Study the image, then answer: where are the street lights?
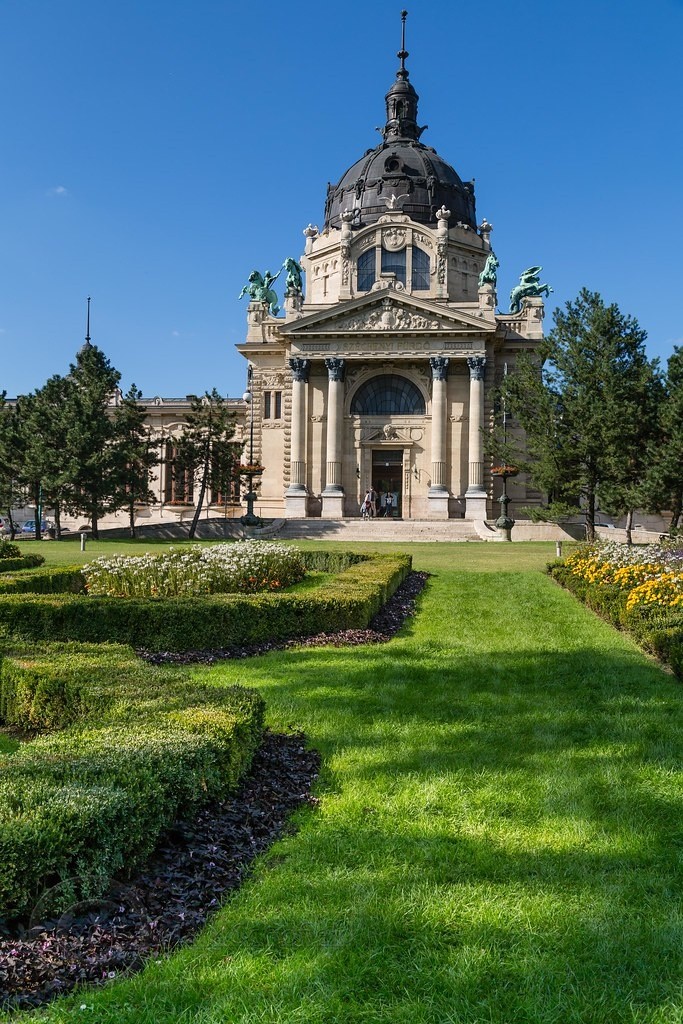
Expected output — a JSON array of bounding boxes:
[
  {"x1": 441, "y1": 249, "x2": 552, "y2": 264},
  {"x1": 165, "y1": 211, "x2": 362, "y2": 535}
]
[{"x1": 242, "y1": 364, "x2": 260, "y2": 526}]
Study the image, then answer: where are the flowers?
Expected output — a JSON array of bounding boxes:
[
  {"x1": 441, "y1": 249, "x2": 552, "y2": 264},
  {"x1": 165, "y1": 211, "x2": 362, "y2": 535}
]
[
  {"x1": 490, "y1": 466, "x2": 517, "y2": 476},
  {"x1": 210, "y1": 500, "x2": 242, "y2": 506},
  {"x1": 240, "y1": 464, "x2": 266, "y2": 471},
  {"x1": 162, "y1": 500, "x2": 195, "y2": 506}
]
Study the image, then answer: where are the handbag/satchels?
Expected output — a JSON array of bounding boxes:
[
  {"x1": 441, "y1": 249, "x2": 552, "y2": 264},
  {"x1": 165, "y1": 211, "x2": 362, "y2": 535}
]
[{"x1": 366, "y1": 501, "x2": 371, "y2": 508}]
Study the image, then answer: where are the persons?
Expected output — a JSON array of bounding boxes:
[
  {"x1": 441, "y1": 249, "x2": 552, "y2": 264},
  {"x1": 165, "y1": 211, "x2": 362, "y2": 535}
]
[
  {"x1": 361, "y1": 490, "x2": 370, "y2": 517},
  {"x1": 384, "y1": 491, "x2": 394, "y2": 517},
  {"x1": 264, "y1": 270, "x2": 281, "y2": 288},
  {"x1": 369, "y1": 486, "x2": 378, "y2": 516}
]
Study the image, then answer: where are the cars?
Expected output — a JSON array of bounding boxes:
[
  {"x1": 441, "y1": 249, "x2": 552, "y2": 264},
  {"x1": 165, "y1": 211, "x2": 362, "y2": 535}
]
[
  {"x1": 0, "y1": 517, "x2": 23, "y2": 535},
  {"x1": 22, "y1": 520, "x2": 55, "y2": 532}
]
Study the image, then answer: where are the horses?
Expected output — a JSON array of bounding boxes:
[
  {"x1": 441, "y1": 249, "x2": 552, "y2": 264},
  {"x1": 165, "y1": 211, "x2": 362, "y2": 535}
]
[
  {"x1": 239, "y1": 271, "x2": 278, "y2": 315},
  {"x1": 509, "y1": 266, "x2": 550, "y2": 312},
  {"x1": 282, "y1": 258, "x2": 302, "y2": 297},
  {"x1": 478, "y1": 250, "x2": 499, "y2": 289}
]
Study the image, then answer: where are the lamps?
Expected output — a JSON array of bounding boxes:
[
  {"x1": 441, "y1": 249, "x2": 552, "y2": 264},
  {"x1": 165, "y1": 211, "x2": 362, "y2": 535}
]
[
  {"x1": 355, "y1": 468, "x2": 360, "y2": 478},
  {"x1": 414, "y1": 469, "x2": 418, "y2": 479}
]
